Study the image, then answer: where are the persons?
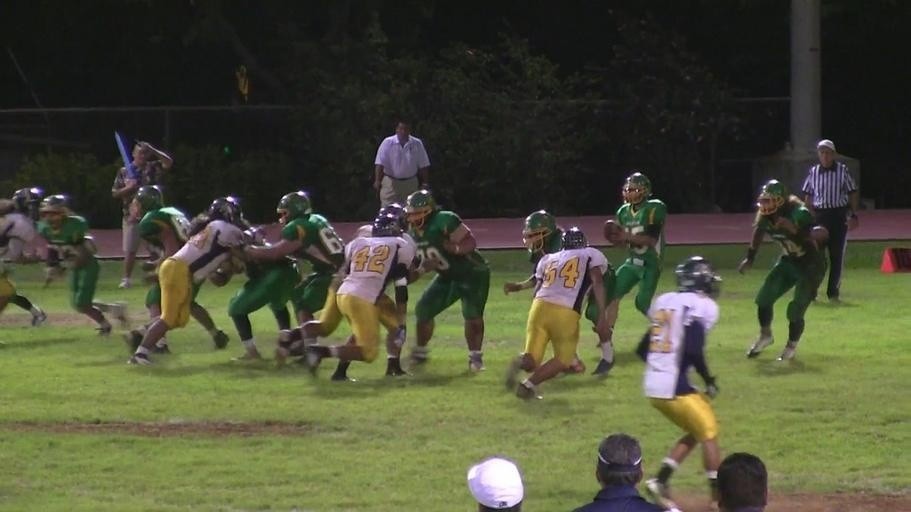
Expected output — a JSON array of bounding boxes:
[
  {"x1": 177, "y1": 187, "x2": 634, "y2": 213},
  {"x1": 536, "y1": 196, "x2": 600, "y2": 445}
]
[
  {"x1": 584, "y1": 171, "x2": 668, "y2": 356},
  {"x1": 375, "y1": 118, "x2": 433, "y2": 212},
  {"x1": 1, "y1": 186, "x2": 128, "y2": 335},
  {"x1": 573, "y1": 432, "x2": 668, "y2": 511},
  {"x1": 737, "y1": 178, "x2": 830, "y2": 362},
  {"x1": 636, "y1": 253, "x2": 722, "y2": 511},
  {"x1": 111, "y1": 140, "x2": 175, "y2": 289},
  {"x1": 504, "y1": 209, "x2": 612, "y2": 402},
  {"x1": 805, "y1": 139, "x2": 859, "y2": 304},
  {"x1": 675, "y1": 502, "x2": 678, "y2": 503},
  {"x1": 122, "y1": 185, "x2": 490, "y2": 382},
  {"x1": 715, "y1": 452, "x2": 769, "y2": 511}
]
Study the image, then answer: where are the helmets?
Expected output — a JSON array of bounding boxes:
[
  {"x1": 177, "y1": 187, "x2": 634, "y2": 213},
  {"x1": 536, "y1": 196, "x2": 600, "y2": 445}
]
[
  {"x1": 406, "y1": 188, "x2": 436, "y2": 216},
  {"x1": 206, "y1": 195, "x2": 244, "y2": 229},
  {"x1": 625, "y1": 170, "x2": 654, "y2": 200},
  {"x1": 133, "y1": 184, "x2": 165, "y2": 212},
  {"x1": 674, "y1": 254, "x2": 717, "y2": 295},
  {"x1": 370, "y1": 202, "x2": 410, "y2": 238},
  {"x1": 762, "y1": 177, "x2": 790, "y2": 207},
  {"x1": 276, "y1": 190, "x2": 311, "y2": 229},
  {"x1": 523, "y1": 208, "x2": 557, "y2": 243},
  {"x1": 563, "y1": 225, "x2": 588, "y2": 250},
  {"x1": 11, "y1": 186, "x2": 71, "y2": 222}
]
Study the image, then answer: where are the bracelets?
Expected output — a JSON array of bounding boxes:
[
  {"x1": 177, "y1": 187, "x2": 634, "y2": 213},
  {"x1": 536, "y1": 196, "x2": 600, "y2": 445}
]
[{"x1": 850, "y1": 212, "x2": 858, "y2": 220}]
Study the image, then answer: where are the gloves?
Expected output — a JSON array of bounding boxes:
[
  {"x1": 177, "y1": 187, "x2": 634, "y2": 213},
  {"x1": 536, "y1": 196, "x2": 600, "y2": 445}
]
[
  {"x1": 737, "y1": 246, "x2": 758, "y2": 275},
  {"x1": 702, "y1": 374, "x2": 721, "y2": 401},
  {"x1": 502, "y1": 280, "x2": 526, "y2": 297},
  {"x1": 408, "y1": 239, "x2": 465, "y2": 275}
]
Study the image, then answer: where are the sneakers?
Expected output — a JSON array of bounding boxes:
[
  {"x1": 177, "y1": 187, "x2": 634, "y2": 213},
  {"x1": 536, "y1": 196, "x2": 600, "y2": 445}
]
[
  {"x1": 502, "y1": 338, "x2": 617, "y2": 401},
  {"x1": 776, "y1": 341, "x2": 798, "y2": 361},
  {"x1": 114, "y1": 299, "x2": 131, "y2": 331},
  {"x1": 233, "y1": 349, "x2": 259, "y2": 360},
  {"x1": 382, "y1": 368, "x2": 413, "y2": 378},
  {"x1": 94, "y1": 323, "x2": 112, "y2": 338},
  {"x1": 468, "y1": 353, "x2": 481, "y2": 373},
  {"x1": 708, "y1": 499, "x2": 723, "y2": 512},
  {"x1": 118, "y1": 276, "x2": 132, "y2": 290},
  {"x1": 409, "y1": 347, "x2": 427, "y2": 364},
  {"x1": 808, "y1": 296, "x2": 845, "y2": 307},
  {"x1": 273, "y1": 329, "x2": 356, "y2": 382},
  {"x1": 126, "y1": 329, "x2": 143, "y2": 348},
  {"x1": 746, "y1": 330, "x2": 774, "y2": 359},
  {"x1": 212, "y1": 331, "x2": 229, "y2": 349},
  {"x1": 154, "y1": 339, "x2": 168, "y2": 352},
  {"x1": 126, "y1": 354, "x2": 155, "y2": 367},
  {"x1": 26, "y1": 307, "x2": 48, "y2": 331},
  {"x1": 642, "y1": 476, "x2": 680, "y2": 512}
]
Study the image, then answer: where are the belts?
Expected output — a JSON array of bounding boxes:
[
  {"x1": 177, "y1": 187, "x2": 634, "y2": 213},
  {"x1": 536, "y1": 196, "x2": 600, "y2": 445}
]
[{"x1": 383, "y1": 174, "x2": 418, "y2": 181}]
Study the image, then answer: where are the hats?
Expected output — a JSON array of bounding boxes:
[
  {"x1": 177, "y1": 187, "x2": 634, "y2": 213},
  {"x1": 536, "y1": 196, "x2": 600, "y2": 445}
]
[{"x1": 816, "y1": 138, "x2": 837, "y2": 155}]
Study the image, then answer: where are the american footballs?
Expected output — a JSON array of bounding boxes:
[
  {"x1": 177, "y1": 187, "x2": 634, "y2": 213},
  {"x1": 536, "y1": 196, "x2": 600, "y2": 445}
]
[{"x1": 602, "y1": 220, "x2": 621, "y2": 244}]
